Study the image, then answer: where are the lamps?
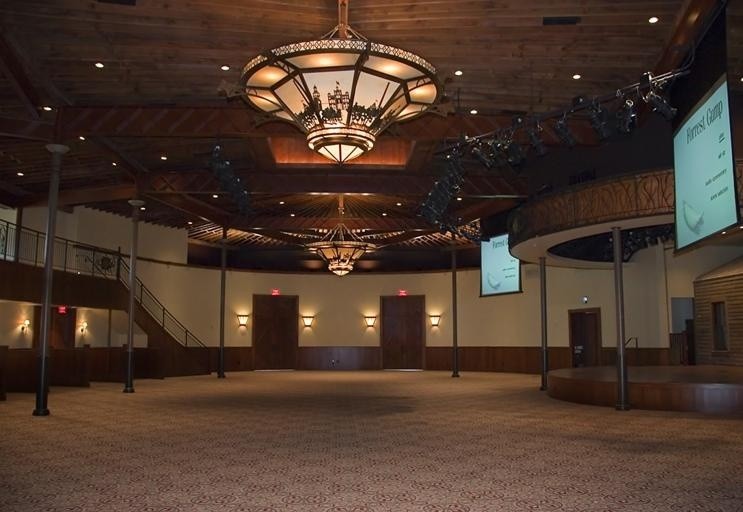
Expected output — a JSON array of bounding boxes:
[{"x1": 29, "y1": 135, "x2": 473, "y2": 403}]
[
  {"x1": 429, "y1": 314, "x2": 440, "y2": 327},
  {"x1": 581, "y1": 296, "x2": 589, "y2": 305},
  {"x1": 303, "y1": 192, "x2": 377, "y2": 277},
  {"x1": 237, "y1": 314, "x2": 249, "y2": 327},
  {"x1": 365, "y1": 317, "x2": 376, "y2": 327},
  {"x1": 19, "y1": 320, "x2": 30, "y2": 335},
  {"x1": 238, "y1": 12, "x2": 446, "y2": 168},
  {"x1": 302, "y1": 316, "x2": 315, "y2": 327},
  {"x1": 80, "y1": 321, "x2": 88, "y2": 333}
]
